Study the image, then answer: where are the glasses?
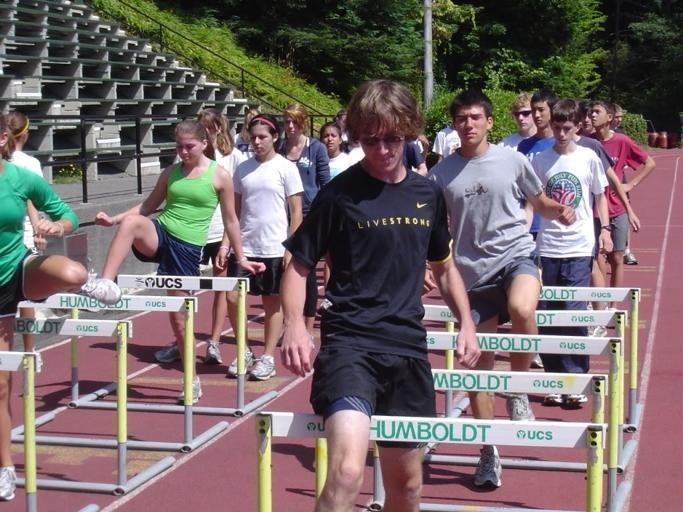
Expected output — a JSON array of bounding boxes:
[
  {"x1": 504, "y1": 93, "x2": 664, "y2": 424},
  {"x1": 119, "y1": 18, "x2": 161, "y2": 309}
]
[
  {"x1": 361, "y1": 133, "x2": 403, "y2": 148},
  {"x1": 514, "y1": 111, "x2": 532, "y2": 116}
]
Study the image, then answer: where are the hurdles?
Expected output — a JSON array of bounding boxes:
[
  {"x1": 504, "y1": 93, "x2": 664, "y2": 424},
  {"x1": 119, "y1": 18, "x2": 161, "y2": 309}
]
[
  {"x1": 10, "y1": 316, "x2": 178, "y2": 495},
  {"x1": 368, "y1": 330, "x2": 635, "y2": 512},
  {"x1": 537, "y1": 284, "x2": 642, "y2": 435},
  {"x1": 1, "y1": 350, "x2": 101, "y2": 512},
  {"x1": 420, "y1": 310, "x2": 638, "y2": 474},
  {"x1": 310, "y1": 365, "x2": 610, "y2": 511},
  {"x1": 69, "y1": 273, "x2": 277, "y2": 419},
  {"x1": 10, "y1": 293, "x2": 231, "y2": 455},
  {"x1": 422, "y1": 301, "x2": 473, "y2": 419},
  {"x1": 255, "y1": 409, "x2": 606, "y2": 512}
]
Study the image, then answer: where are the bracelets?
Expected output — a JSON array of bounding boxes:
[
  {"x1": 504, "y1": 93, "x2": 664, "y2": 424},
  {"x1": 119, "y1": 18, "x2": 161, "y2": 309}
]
[{"x1": 55, "y1": 222, "x2": 67, "y2": 241}]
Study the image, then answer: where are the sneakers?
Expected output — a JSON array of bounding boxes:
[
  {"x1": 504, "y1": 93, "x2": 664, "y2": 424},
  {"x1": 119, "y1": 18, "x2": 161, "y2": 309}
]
[
  {"x1": 0, "y1": 465, "x2": 18, "y2": 501},
  {"x1": 319, "y1": 297, "x2": 333, "y2": 311},
  {"x1": 278, "y1": 332, "x2": 315, "y2": 350},
  {"x1": 623, "y1": 251, "x2": 637, "y2": 265},
  {"x1": 505, "y1": 393, "x2": 534, "y2": 423},
  {"x1": 71, "y1": 271, "x2": 121, "y2": 304},
  {"x1": 586, "y1": 326, "x2": 607, "y2": 338},
  {"x1": 205, "y1": 338, "x2": 223, "y2": 363},
  {"x1": 155, "y1": 342, "x2": 183, "y2": 363},
  {"x1": 543, "y1": 390, "x2": 587, "y2": 406},
  {"x1": 531, "y1": 354, "x2": 545, "y2": 368},
  {"x1": 228, "y1": 347, "x2": 276, "y2": 381},
  {"x1": 474, "y1": 442, "x2": 502, "y2": 488},
  {"x1": 178, "y1": 375, "x2": 203, "y2": 404}
]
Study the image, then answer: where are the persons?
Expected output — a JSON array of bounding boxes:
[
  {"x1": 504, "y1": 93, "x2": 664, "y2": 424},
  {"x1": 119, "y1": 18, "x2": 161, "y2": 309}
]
[
  {"x1": 402, "y1": 88, "x2": 657, "y2": 491},
  {"x1": 279, "y1": 78, "x2": 481, "y2": 512},
  {"x1": 96, "y1": 105, "x2": 365, "y2": 404},
  {"x1": 4, "y1": 112, "x2": 47, "y2": 259},
  {"x1": 0, "y1": 122, "x2": 121, "y2": 504}
]
